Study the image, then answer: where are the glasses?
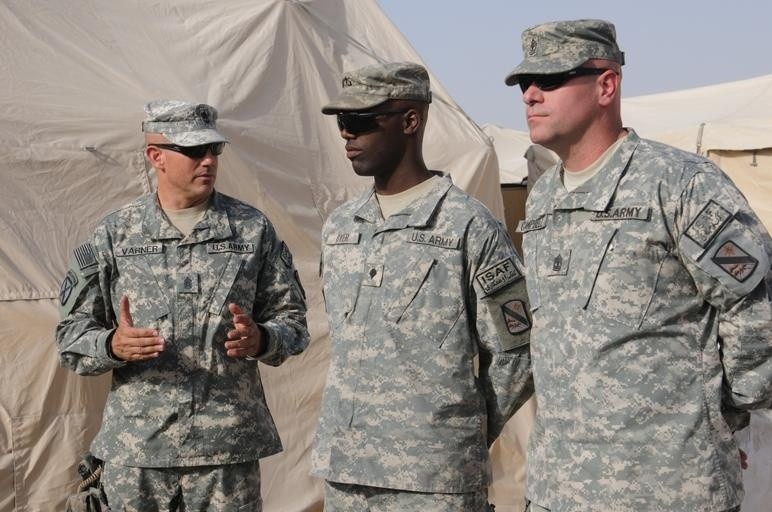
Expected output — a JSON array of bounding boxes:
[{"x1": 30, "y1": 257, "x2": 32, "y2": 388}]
[
  {"x1": 520, "y1": 67, "x2": 620, "y2": 95},
  {"x1": 337, "y1": 109, "x2": 425, "y2": 134},
  {"x1": 148, "y1": 143, "x2": 225, "y2": 158}
]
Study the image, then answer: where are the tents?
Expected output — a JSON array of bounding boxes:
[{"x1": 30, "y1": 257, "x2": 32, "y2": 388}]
[
  {"x1": 478, "y1": 121, "x2": 541, "y2": 264},
  {"x1": 0, "y1": 0, "x2": 533, "y2": 512},
  {"x1": 617, "y1": 73, "x2": 772, "y2": 242}
]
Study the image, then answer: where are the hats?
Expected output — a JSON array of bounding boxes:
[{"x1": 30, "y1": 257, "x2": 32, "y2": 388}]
[
  {"x1": 321, "y1": 61, "x2": 433, "y2": 115},
  {"x1": 141, "y1": 98, "x2": 231, "y2": 147},
  {"x1": 504, "y1": 19, "x2": 626, "y2": 87}
]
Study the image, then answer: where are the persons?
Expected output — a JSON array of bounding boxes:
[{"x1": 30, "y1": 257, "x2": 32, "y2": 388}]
[
  {"x1": 50, "y1": 96, "x2": 313, "y2": 512},
  {"x1": 303, "y1": 58, "x2": 535, "y2": 512},
  {"x1": 506, "y1": 15, "x2": 771, "y2": 512}
]
